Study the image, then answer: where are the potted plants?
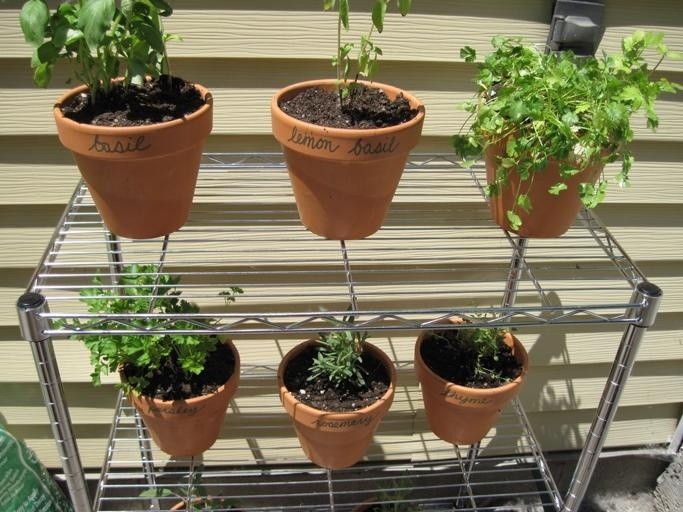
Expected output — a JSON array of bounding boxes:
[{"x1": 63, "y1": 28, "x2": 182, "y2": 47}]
[
  {"x1": 445, "y1": 27, "x2": 682, "y2": 243},
  {"x1": 348, "y1": 471, "x2": 421, "y2": 512},
  {"x1": 275, "y1": 300, "x2": 397, "y2": 468},
  {"x1": 19, "y1": 0, "x2": 215, "y2": 240},
  {"x1": 52, "y1": 263, "x2": 241, "y2": 457},
  {"x1": 412, "y1": 300, "x2": 530, "y2": 444},
  {"x1": 268, "y1": 1, "x2": 427, "y2": 241},
  {"x1": 137, "y1": 467, "x2": 242, "y2": 510}
]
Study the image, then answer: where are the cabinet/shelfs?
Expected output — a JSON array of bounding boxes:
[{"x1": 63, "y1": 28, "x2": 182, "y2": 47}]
[{"x1": 13, "y1": 152, "x2": 664, "y2": 510}]
